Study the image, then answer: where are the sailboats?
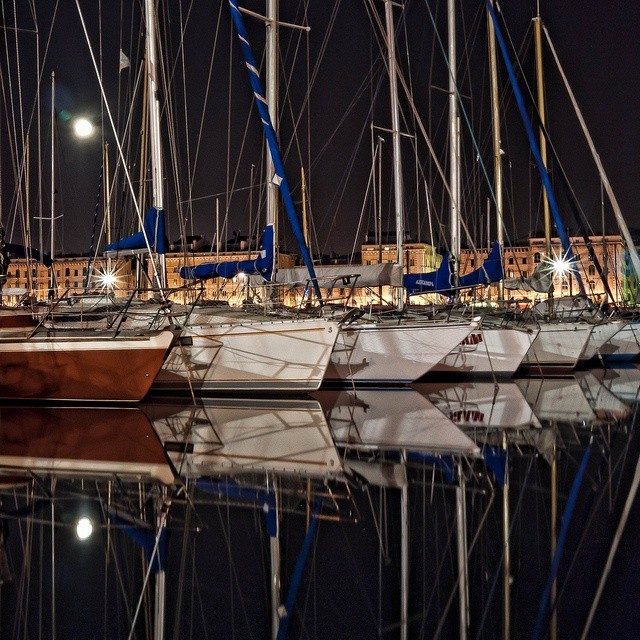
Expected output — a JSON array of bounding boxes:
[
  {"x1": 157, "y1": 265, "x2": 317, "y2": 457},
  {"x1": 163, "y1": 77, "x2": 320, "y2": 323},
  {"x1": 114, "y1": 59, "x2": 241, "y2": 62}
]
[
  {"x1": 1, "y1": 0, "x2": 640, "y2": 408},
  {"x1": 0, "y1": 362, "x2": 640, "y2": 638},
  {"x1": 2, "y1": 367, "x2": 638, "y2": 639}
]
